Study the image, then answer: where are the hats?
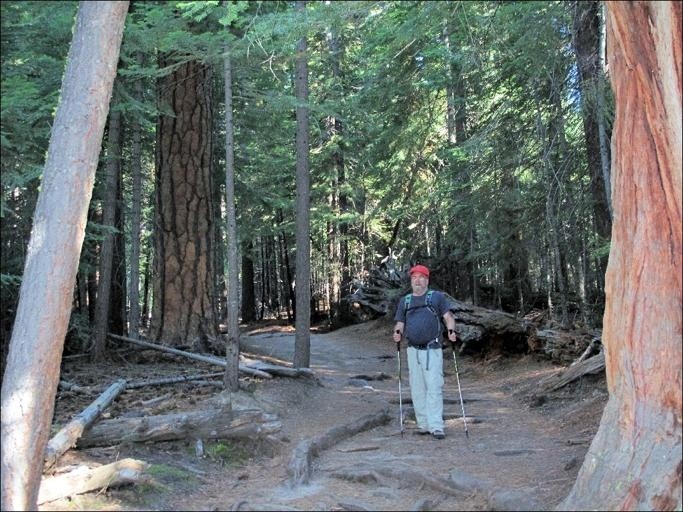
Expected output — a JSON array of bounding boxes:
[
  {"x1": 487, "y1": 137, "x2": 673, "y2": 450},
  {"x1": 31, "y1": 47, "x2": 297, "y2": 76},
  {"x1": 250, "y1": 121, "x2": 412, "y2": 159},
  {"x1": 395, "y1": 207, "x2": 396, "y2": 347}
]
[{"x1": 410, "y1": 265, "x2": 429, "y2": 276}]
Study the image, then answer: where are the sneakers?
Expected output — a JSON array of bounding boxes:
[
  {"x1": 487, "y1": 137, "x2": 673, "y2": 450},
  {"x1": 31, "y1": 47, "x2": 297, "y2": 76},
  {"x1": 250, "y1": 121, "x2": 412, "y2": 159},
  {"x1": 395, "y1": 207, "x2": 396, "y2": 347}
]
[
  {"x1": 433, "y1": 430, "x2": 445, "y2": 439},
  {"x1": 413, "y1": 427, "x2": 430, "y2": 435}
]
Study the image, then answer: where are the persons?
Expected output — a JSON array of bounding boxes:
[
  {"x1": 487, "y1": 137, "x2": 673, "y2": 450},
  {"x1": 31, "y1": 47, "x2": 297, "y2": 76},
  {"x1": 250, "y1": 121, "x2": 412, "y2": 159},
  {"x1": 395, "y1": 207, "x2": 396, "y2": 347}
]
[{"x1": 392, "y1": 265, "x2": 457, "y2": 440}]
